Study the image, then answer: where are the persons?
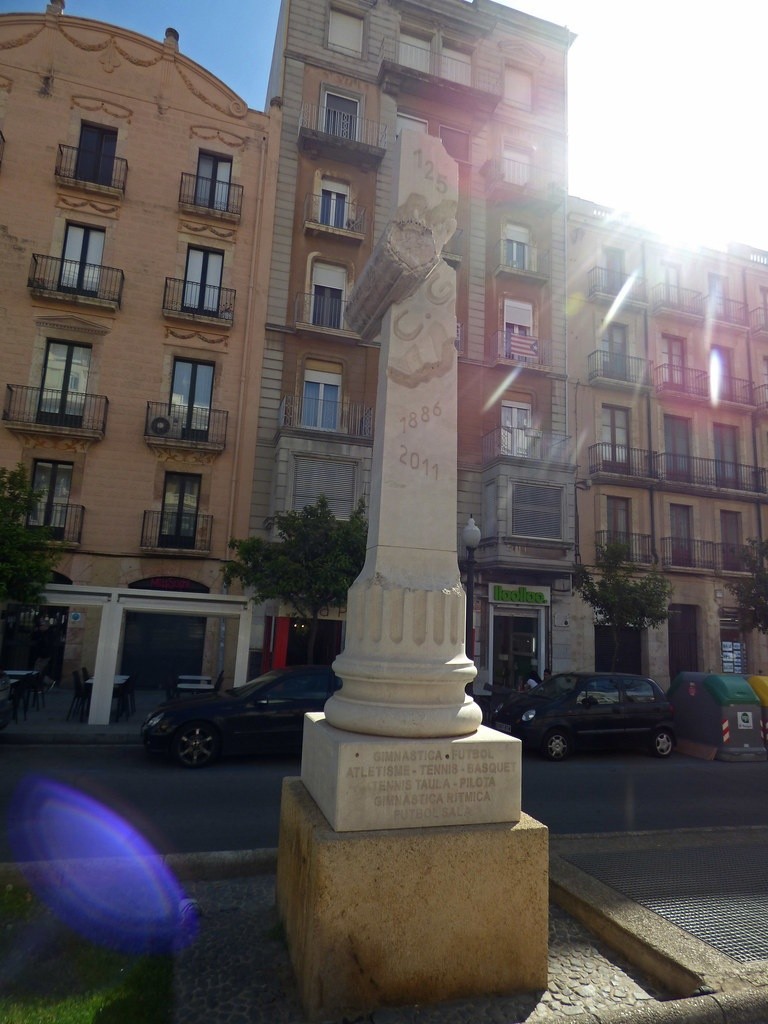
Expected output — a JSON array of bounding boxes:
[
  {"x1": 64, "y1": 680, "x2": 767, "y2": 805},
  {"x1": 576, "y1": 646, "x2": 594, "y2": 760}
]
[{"x1": 525, "y1": 671, "x2": 542, "y2": 692}]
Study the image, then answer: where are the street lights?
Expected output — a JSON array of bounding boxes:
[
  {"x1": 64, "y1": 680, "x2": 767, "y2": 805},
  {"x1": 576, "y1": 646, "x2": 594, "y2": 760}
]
[{"x1": 462, "y1": 513, "x2": 480, "y2": 698}]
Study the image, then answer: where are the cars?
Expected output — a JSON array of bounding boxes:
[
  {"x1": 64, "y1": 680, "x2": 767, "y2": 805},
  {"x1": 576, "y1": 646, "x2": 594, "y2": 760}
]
[
  {"x1": 137, "y1": 664, "x2": 342, "y2": 770},
  {"x1": 490, "y1": 669, "x2": 678, "y2": 758}
]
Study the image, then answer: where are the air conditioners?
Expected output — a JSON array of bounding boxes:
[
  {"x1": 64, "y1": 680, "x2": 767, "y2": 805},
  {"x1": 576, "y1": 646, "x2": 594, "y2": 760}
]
[{"x1": 146, "y1": 415, "x2": 184, "y2": 439}]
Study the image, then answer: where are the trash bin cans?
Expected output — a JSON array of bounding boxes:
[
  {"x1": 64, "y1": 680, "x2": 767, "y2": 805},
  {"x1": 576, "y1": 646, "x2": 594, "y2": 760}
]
[{"x1": 670, "y1": 672, "x2": 768, "y2": 762}]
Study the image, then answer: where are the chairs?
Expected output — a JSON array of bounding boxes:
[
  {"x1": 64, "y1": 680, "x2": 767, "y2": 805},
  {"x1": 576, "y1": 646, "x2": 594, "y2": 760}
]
[{"x1": 0, "y1": 666, "x2": 226, "y2": 726}]
